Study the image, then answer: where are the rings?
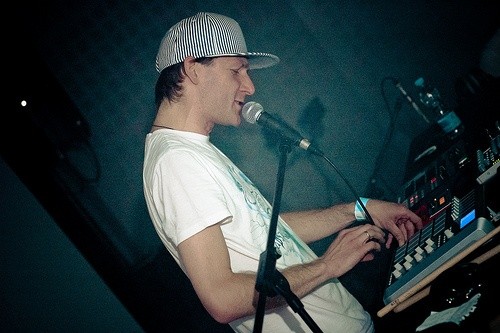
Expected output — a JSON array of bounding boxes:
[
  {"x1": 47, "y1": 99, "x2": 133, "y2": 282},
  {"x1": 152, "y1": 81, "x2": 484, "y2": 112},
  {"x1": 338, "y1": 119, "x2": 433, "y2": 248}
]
[{"x1": 364, "y1": 230, "x2": 371, "y2": 241}]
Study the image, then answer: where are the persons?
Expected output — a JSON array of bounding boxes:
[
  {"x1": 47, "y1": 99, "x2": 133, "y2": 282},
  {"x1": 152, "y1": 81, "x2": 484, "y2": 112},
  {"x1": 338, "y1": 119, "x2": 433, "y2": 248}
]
[{"x1": 143, "y1": 12, "x2": 423, "y2": 332}]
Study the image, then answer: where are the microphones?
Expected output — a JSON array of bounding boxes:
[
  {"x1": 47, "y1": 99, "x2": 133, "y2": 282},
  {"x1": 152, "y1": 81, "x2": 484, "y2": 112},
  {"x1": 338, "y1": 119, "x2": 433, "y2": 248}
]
[
  {"x1": 394, "y1": 79, "x2": 434, "y2": 126},
  {"x1": 241, "y1": 101, "x2": 325, "y2": 157}
]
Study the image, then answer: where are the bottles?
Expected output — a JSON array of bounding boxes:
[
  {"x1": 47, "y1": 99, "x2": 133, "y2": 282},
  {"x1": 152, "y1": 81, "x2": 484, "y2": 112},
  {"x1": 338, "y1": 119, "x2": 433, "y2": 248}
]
[{"x1": 415, "y1": 77, "x2": 465, "y2": 140}]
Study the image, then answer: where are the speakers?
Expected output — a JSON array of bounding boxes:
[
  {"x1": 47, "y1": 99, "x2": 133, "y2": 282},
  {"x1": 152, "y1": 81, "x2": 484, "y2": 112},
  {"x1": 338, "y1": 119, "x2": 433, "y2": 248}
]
[{"x1": 29, "y1": 80, "x2": 91, "y2": 152}]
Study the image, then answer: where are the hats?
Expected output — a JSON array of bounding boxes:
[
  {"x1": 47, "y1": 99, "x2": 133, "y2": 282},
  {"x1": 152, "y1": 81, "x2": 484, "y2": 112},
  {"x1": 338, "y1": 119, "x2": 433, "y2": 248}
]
[{"x1": 155, "y1": 12, "x2": 281, "y2": 72}]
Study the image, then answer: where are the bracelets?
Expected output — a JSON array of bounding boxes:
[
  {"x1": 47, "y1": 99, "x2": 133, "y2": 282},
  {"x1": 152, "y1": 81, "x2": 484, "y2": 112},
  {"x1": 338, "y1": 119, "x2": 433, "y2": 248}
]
[{"x1": 354, "y1": 197, "x2": 370, "y2": 221}]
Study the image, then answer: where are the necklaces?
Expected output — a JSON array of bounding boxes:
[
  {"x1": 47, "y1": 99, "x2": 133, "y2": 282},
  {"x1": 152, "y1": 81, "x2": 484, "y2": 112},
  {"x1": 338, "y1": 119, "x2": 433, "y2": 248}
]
[{"x1": 152, "y1": 124, "x2": 173, "y2": 129}]
